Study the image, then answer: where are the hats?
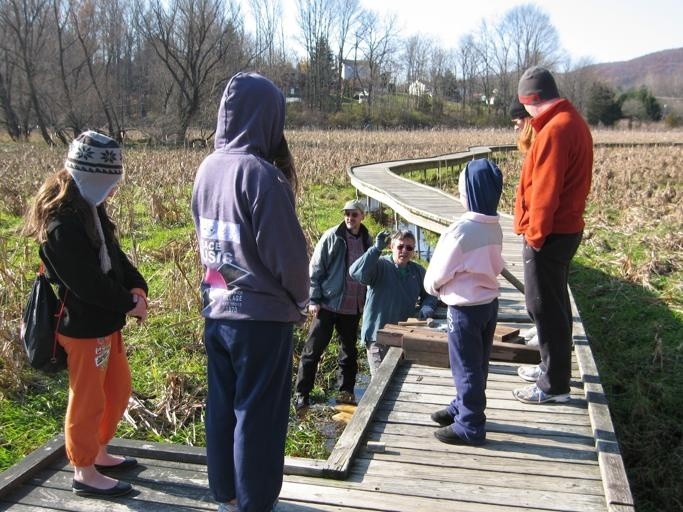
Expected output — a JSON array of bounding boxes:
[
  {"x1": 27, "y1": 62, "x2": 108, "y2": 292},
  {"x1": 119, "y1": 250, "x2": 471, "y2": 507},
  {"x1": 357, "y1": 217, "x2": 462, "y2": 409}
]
[
  {"x1": 519, "y1": 65, "x2": 559, "y2": 105},
  {"x1": 342, "y1": 199, "x2": 363, "y2": 211},
  {"x1": 66, "y1": 130, "x2": 124, "y2": 205}
]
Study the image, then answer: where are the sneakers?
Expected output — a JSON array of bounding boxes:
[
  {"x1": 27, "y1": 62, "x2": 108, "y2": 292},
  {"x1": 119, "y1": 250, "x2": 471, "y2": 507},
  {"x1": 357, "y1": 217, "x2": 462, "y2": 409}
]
[
  {"x1": 513, "y1": 383, "x2": 569, "y2": 404},
  {"x1": 431, "y1": 407, "x2": 455, "y2": 424},
  {"x1": 72, "y1": 476, "x2": 133, "y2": 497},
  {"x1": 93, "y1": 455, "x2": 137, "y2": 471},
  {"x1": 434, "y1": 423, "x2": 486, "y2": 445},
  {"x1": 517, "y1": 363, "x2": 547, "y2": 381}
]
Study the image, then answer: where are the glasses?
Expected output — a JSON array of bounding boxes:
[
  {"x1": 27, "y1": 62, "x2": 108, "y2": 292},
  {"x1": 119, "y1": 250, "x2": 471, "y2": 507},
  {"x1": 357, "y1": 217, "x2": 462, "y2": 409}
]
[
  {"x1": 344, "y1": 212, "x2": 358, "y2": 217},
  {"x1": 397, "y1": 244, "x2": 414, "y2": 252}
]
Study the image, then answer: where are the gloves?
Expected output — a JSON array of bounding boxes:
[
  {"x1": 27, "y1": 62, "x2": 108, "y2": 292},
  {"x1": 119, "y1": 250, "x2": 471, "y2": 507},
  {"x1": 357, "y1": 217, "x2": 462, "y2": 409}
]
[
  {"x1": 418, "y1": 304, "x2": 433, "y2": 320},
  {"x1": 376, "y1": 230, "x2": 390, "y2": 250}
]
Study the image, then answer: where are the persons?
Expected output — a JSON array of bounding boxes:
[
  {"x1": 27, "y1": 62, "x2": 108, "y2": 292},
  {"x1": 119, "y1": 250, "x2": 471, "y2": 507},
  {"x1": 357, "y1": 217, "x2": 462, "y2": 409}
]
[
  {"x1": 20, "y1": 129, "x2": 149, "y2": 498},
  {"x1": 191, "y1": 70, "x2": 312, "y2": 511},
  {"x1": 422, "y1": 157, "x2": 506, "y2": 446},
  {"x1": 510, "y1": 96, "x2": 539, "y2": 346},
  {"x1": 293, "y1": 200, "x2": 374, "y2": 405},
  {"x1": 512, "y1": 65, "x2": 594, "y2": 402},
  {"x1": 348, "y1": 228, "x2": 441, "y2": 376}
]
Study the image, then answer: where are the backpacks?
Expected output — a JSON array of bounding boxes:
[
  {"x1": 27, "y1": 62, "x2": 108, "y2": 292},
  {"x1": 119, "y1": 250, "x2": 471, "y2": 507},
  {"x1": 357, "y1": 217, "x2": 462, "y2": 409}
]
[{"x1": 19, "y1": 273, "x2": 69, "y2": 374}]
